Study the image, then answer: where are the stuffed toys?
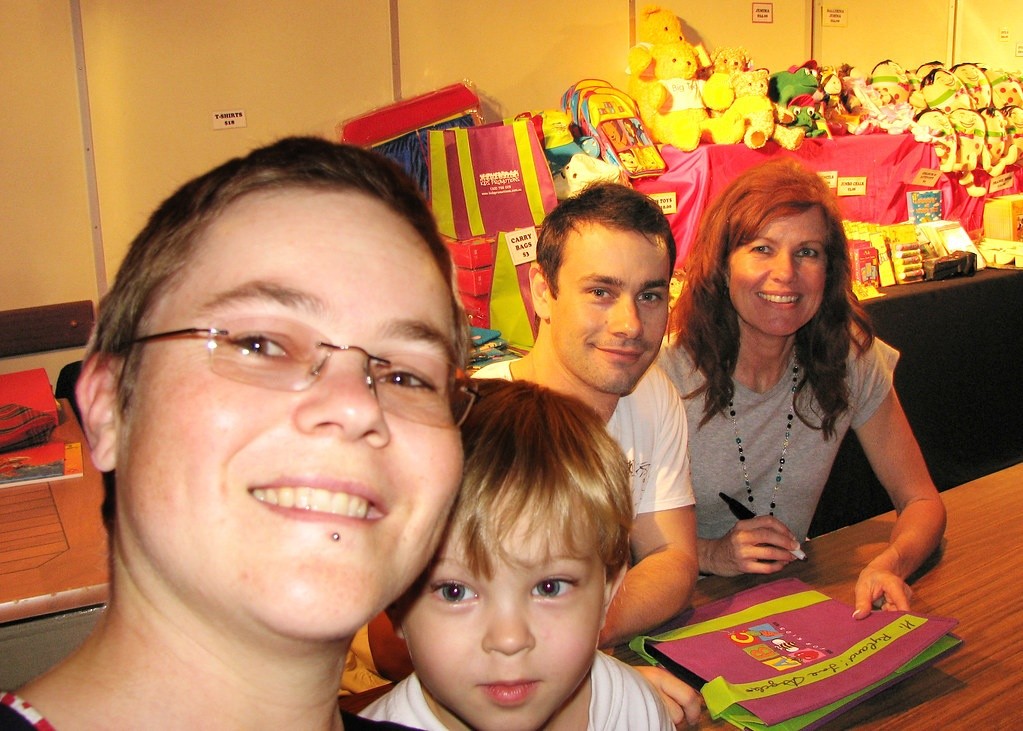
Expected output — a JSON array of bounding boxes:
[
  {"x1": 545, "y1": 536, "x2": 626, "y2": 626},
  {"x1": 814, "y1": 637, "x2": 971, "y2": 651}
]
[
  {"x1": 626, "y1": 8, "x2": 1023, "y2": 197},
  {"x1": 914, "y1": 108, "x2": 967, "y2": 174},
  {"x1": 871, "y1": 61, "x2": 910, "y2": 103},
  {"x1": 921, "y1": 68, "x2": 976, "y2": 113},
  {"x1": 729, "y1": 70, "x2": 803, "y2": 150}
]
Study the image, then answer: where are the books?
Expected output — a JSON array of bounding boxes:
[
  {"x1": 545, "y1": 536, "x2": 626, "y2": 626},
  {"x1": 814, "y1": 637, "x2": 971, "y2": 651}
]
[{"x1": 1, "y1": 439, "x2": 84, "y2": 489}]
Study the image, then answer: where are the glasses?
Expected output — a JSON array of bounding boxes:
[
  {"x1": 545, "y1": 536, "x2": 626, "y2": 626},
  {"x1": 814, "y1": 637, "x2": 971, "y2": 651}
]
[{"x1": 109, "y1": 327, "x2": 479, "y2": 429}]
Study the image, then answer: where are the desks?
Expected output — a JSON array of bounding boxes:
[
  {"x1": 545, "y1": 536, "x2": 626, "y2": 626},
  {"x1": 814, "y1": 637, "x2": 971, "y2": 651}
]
[{"x1": 338, "y1": 463, "x2": 1023, "y2": 731}]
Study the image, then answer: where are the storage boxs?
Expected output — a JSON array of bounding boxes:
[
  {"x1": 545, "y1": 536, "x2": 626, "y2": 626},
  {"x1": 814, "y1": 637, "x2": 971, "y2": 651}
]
[
  {"x1": 0, "y1": 366, "x2": 59, "y2": 426},
  {"x1": 439, "y1": 235, "x2": 496, "y2": 330},
  {"x1": 982, "y1": 194, "x2": 1023, "y2": 243}
]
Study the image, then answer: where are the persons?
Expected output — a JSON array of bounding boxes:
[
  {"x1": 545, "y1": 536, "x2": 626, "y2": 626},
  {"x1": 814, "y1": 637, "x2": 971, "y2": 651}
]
[
  {"x1": 361, "y1": 379, "x2": 686, "y2": 731},
  {"x1": 0, "y1": 138, "x2": 474, "y2": 731},
  {"x1": 467, "y1": 180, "x2": 696, "y2": 646},
  {"x1": 654, "y1": 159, "x2": 947, "y2": 620}
]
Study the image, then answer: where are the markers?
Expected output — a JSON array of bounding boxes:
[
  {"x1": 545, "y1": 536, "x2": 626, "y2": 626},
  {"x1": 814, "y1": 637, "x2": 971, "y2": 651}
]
[{"x1": 717, "y1": 490, "x2": 809, "y2": 562}]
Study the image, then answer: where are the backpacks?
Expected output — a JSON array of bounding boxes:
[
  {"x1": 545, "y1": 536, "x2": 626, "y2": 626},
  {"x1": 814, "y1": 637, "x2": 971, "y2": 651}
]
[{"x1": 561, "y1": 78, "x2": 667, "y2": 180}]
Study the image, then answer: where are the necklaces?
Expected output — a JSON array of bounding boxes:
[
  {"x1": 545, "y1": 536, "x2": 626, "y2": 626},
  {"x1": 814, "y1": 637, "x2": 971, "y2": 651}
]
[{"x1": 728, "y1": 366, "x2": 798, "y2": 517}]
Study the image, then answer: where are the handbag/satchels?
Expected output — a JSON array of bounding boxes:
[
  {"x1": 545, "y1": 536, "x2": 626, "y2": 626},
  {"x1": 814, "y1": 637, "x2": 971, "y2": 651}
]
[
  {"x1": 627, "y1": 575, "x2": 964, "y2": 731},
  {"x1": 427, "y1": 116, "x2": 559, "y2": 240}
]
